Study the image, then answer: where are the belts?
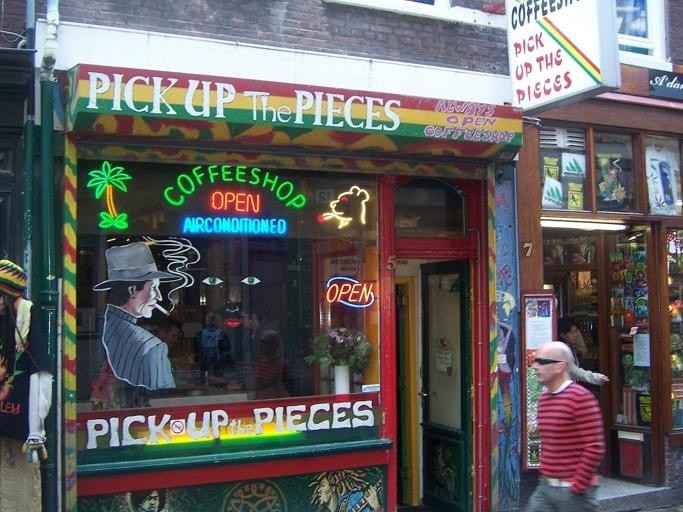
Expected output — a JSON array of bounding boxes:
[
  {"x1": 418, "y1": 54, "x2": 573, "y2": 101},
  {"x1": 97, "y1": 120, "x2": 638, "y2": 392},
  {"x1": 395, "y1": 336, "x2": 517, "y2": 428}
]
[{"x1": 546, "y1": 478, "x2": 574, "y2": 488}]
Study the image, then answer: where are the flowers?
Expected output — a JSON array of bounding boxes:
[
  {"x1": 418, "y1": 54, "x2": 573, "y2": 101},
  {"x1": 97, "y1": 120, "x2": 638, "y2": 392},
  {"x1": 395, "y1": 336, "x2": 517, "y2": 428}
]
[{"x1": 303, "y1": 327, "x2": 374, "y2": 376}]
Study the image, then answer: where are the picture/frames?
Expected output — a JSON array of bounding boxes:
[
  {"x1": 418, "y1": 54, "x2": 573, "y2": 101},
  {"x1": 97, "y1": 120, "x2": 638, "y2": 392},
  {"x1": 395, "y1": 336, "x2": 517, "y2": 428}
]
[{"x1": 519, "y1": 292, "x2": 558, "y2": 470}]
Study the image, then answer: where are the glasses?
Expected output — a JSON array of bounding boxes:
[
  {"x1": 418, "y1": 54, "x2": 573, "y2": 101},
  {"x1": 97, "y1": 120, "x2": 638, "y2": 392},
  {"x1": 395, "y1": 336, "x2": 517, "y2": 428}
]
[{"x1": 534, "y1": 357, "x2": 564, "y2": 365}]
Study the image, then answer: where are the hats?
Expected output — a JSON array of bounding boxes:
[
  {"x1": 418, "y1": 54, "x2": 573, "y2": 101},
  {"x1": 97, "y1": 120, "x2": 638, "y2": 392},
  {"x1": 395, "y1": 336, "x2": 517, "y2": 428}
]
[{"x1": 92, "y1": 241, "x2": 182, "y2": 291}]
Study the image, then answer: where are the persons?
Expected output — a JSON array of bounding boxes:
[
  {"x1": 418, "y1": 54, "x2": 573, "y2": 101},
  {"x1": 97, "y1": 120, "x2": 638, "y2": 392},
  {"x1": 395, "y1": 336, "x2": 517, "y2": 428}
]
[
  {"x1": 522, "y1": 341, "x2": 607, "y2": 511},
  {"x1": 0, "y1": 256, "x2": 57, "y2": 459},
  {"x1": 193, "y1": 312, "x2": 231, "y2": 367},
  {"x1": 312, "y1": 470, "x2": 382, "y2": 511},
  {"x1": 89, "y1": 239, "x2": 178, "y2": 392},
  {"x1": 555, "y1": 318, "x2": 610, "y2": 388},
  {"x1": 228, "y1": 300, "x2": 284, "y2": 401},
  {"x1": 133, "y1": 486, "x2": 166, "y2": 512}
]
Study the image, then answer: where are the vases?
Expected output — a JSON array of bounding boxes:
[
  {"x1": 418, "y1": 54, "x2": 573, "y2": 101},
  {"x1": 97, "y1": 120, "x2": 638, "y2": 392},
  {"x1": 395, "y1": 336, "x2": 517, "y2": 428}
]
[{"x1": 333, "y1": 365, "x2": 351, "y2": 394}]
